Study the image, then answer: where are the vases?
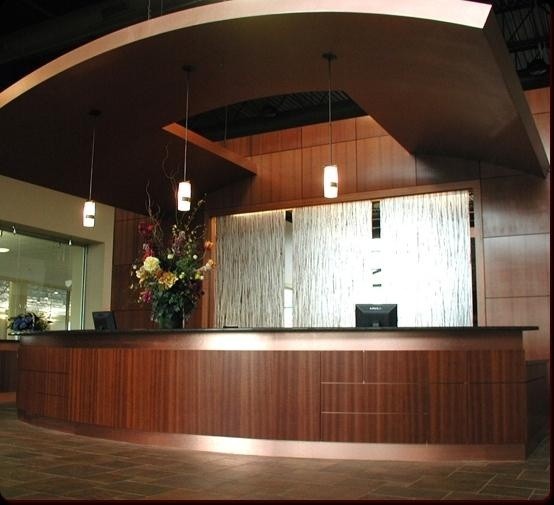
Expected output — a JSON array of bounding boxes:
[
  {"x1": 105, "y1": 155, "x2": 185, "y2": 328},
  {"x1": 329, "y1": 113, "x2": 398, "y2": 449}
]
[{"x1": 157, "y1": 296, "x2": 184, "y2": 331}]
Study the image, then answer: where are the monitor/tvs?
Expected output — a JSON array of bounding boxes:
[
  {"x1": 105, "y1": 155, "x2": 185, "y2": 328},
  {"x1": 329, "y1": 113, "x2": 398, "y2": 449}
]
[
  {"x1": 355, "y1": 304, "x2": 398, "y2": 327},
  {"x1": 92, "y1": 311, "x2": 117, "y2": 330}
]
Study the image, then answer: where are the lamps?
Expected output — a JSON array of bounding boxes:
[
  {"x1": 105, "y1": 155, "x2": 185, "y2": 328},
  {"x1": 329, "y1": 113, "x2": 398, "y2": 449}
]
[
  {"x1": 175, "y1": 66, "x2": 196, "y2": 212},
  {"x1": 80, "y1": 107, "x2": 100, "y2": 229},
  {"x1": 321, "y1": 53, "x2": 340, "y2": 198}
]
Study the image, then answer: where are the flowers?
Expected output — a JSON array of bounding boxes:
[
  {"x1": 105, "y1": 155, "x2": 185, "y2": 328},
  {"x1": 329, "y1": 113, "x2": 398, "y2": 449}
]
[
  {"x1": 127, "y1": 175, "x2": 214, "y2": 326},
  {"x1": 5, "y1": 311, "x2": 52, "y2": 334}
]
